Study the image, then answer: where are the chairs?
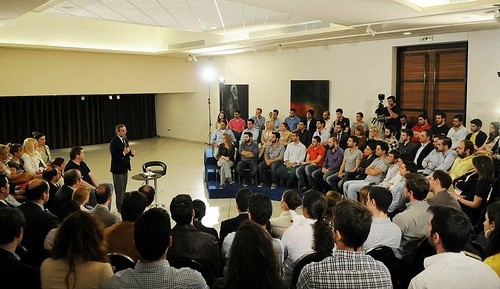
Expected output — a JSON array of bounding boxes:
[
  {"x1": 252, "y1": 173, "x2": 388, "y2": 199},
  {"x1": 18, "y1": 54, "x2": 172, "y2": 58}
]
[
  {"x1": 293, "y1": 236, "x2": 436, "y2": 289},
  {"x1": 107, "y1": 253, "x2": 213, "y2": 289}
]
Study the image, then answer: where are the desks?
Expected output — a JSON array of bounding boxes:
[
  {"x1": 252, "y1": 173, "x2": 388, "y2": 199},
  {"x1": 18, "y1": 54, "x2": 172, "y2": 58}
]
[{"x1": 132, "y1": 173, "x2": 161, "y2": 207}]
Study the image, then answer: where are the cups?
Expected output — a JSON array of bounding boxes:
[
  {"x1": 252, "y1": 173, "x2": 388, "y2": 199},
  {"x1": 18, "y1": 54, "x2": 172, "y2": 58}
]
[{"x1": 146, "y1": 167, "x2": 151, "y2": 172}]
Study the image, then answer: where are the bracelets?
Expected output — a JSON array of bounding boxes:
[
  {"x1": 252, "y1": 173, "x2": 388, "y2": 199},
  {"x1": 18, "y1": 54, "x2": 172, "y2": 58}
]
[{"x1": 457, "y1": 197, "x2": 461, "y2": 201}]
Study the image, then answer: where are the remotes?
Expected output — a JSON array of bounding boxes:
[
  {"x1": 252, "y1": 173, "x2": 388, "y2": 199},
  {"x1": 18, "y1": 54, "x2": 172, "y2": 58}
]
[{"x1": 139, "y1": 173, "x2": 148, "y2": 178}]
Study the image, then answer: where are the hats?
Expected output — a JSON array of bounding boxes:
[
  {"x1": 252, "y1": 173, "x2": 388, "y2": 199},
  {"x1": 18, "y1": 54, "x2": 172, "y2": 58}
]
[{"x1": 491, "y1": 121, "x2": 500, "y2": 130}]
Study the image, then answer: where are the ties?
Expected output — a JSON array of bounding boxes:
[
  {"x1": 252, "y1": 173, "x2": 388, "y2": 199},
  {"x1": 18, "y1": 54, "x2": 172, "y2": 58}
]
[{"x1": 122, "y1": 139, "x2": 128, "y2": 148}]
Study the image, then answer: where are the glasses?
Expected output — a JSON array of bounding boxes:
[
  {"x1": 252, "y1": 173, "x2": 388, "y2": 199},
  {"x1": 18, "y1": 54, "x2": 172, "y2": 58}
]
[{"x1": 119, "y1": 130, "x2": 127, "y2": 133}]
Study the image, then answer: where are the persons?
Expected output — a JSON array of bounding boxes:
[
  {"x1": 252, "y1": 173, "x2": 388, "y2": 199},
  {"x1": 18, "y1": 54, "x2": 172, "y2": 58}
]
[
  {"x1": 383, "y1": 96, "x2": 400, "y2": 131},
  {"x1": 110, "y1": 125, "x2": 134, "y2": 214},
  {"x1": 0, "y1": 132, "x2": 224, "y2": 289},
  {"x1": 213, "y1": 108, "x2": 500, "y2": 289}
]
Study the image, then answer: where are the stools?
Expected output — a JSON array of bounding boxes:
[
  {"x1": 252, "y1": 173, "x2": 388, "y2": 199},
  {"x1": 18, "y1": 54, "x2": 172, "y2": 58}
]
[
  {"x1": 142, "y1": 161, "x2": 167, "y2": 207},
  {"x1": 215, "y1": 167, "x2": 300, "y2": 189}
]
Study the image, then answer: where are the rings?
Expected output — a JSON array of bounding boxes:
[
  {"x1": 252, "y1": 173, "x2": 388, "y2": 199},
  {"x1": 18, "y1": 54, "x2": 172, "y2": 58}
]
[{"x1": 451, "y1": 194, "x2": 452, "y2": 196}]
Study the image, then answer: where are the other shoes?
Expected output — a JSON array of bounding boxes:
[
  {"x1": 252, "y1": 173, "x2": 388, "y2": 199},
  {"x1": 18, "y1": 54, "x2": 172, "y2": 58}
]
[
  {"x1": 258, "y1": 182, "x2": 266, "y2": 188},
  {"x1": 271, "y1": 182, "x2": 278, "y2": 190},
  {"x1": 219, "y1": 184, "x2": 225, "y2": 190},
  {"x1": 251, "y1": 178, "x2": 255, "y2": 185},
  {"x1": 298, "y1": 186, "x2": 308, "y2": 192},
  {"x1": 243, "y1": 178, "x2": 248, "y2": 188},
  {"x1": 228, "y1": 178, "x2": 235, "y2": 186}
]
